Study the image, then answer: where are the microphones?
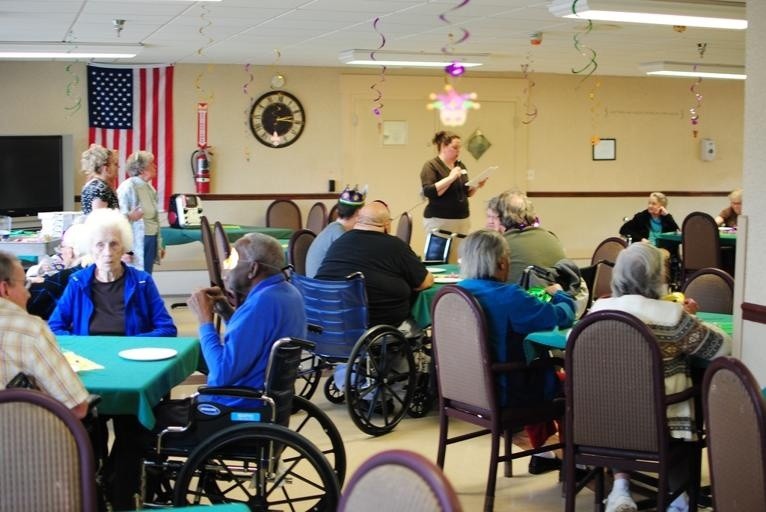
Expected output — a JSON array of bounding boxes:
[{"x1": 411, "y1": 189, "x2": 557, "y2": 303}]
[{"x1": 454, "y1": 159, "x2": 461, "y2": 167}]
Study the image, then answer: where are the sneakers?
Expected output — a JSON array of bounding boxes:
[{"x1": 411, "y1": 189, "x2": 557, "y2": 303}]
[
  {"x1": 528, "y1": 453, "x2": 561, "y2": 474},
  {"x1": 603, "y1": 492, "x2": 637, "y2": 512}
]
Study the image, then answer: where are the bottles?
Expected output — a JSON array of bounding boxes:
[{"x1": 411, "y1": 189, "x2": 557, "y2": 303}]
[{"x1": 626, "y1": 234, "x2": 632, "y2": 245}]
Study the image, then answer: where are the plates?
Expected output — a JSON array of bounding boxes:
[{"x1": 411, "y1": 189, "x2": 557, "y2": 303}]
[
  {"x1": 118, "y1": 346, "x2": 178, "y2": 363},
  {"x1": 717, "y1": 225, "x2": 733, "y2": 232},
  {"x1": 425, "y1": 265, "x2": 463, "y2": 284}
]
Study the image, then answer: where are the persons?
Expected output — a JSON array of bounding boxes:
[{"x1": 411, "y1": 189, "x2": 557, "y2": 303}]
[
  {"x1": 584, "y1": 241, "x2": 732, "y2": 512},
  {"x1": 620, "y1": 193, "x2": 683, "y2": 270},
  {"x1": 455, "y1": 230, "x2": 590, "y2": 484},
  {"x1": 312, "y1": 200, "x2": 435, "y2": 417},
  {"x1": 496, "y1": 188, "x2": 570, "y2": 285},
  {"x1": 486, "y1": 196, "x2": 517, "y2": 234},
  {"x1": 305, "y1": 191, "x2": 367, "y2": 282},
  {"x1": 116, "y1": 151, "x2": 166, "y2": 278},
  {"x1": 0, "y1": 249, "x2": 104, "y2": 433},
  {"x1": 419, "y1": 131, "x2": 490, "y2": 265},
  {"x1": 100, "y1": 231, "x2": 309, "y2": 512},
  {"x1": 28, "y1": 224, "x2": 100, "y2": 320},
  {"x1": 78, "y1": 143, "x2": 120, "y2": 215},
  {"x1": 716, "y1": 189, "x2": 743, "y2": 228},
  {"x1": 47, "y1": 207, "x2": 179, "y2": 512}
]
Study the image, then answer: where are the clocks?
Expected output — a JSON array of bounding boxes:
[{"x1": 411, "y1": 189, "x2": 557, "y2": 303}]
[{"x1": 248, "y1": 89, "x2": 306, "y2": 149}]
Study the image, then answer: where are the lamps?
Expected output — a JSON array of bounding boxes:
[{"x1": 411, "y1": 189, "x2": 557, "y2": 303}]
[
  {"x1": 336, "y1": 50, "x2": 492, "y2": 69},
  {"x1": 547, "y1": 1, "x2": 748, "y2": 80},
  {"x1": 0, "y1": 42, "x2": 143, "y2": 60}
]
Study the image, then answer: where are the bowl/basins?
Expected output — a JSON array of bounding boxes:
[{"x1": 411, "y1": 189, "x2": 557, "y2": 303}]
[{"x1": 0, "y1": 234, "x2": 9, "y2": 241}]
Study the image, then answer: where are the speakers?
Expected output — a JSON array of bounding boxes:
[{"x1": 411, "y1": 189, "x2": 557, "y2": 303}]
[{"x1": 700, "y1": 139, "x2": 715, "y2": 160}]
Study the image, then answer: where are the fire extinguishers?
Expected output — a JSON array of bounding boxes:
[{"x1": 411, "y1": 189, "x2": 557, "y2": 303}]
[{"x1": 191, "y1": 146, "x2": 213, "y2": 193}]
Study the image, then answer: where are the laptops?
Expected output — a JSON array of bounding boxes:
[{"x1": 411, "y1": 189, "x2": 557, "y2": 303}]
[{"x1": 419, "y1": 234, "x2": 452, "y2": 264}]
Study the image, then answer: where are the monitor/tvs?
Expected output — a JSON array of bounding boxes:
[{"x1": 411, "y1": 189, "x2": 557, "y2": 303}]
[{"x1": 0, "y1": 134, "x2": 64, "y2": 216}]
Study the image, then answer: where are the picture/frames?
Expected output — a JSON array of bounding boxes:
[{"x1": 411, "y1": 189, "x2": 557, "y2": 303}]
[{"x1": 592, "y1": 137, "x2": 617, "y2": 162}]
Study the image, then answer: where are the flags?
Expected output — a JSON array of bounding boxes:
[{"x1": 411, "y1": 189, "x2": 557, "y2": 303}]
[{"x1": 85, "y1": 62, "x2": 175, "y2": 215}]
